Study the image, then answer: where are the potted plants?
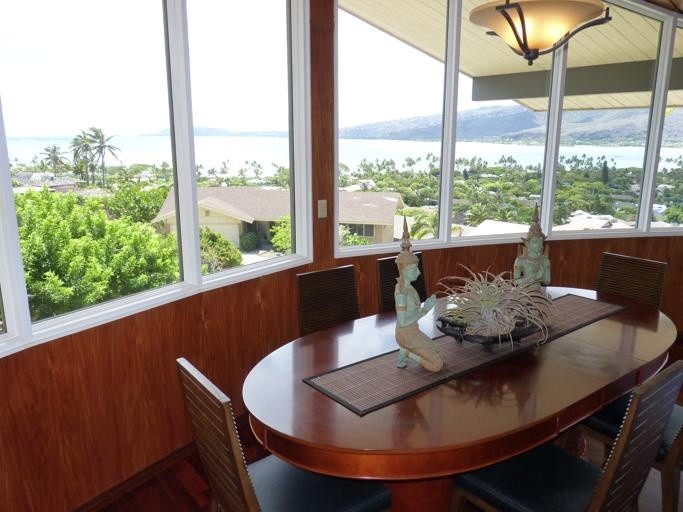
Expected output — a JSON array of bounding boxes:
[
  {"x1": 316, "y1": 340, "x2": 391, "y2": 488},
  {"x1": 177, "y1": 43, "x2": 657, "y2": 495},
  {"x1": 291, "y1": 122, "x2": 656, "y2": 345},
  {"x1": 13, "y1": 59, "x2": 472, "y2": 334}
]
[{"x1": 436, "y1": 262, "x2": 554, "y2": 351}]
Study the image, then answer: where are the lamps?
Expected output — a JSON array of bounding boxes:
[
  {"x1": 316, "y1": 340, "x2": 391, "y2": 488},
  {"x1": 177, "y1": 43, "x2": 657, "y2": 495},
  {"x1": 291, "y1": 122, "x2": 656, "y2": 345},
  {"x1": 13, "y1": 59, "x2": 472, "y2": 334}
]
[{"x1": 468, "y1": 0, "x2": 612, "y2": 66}]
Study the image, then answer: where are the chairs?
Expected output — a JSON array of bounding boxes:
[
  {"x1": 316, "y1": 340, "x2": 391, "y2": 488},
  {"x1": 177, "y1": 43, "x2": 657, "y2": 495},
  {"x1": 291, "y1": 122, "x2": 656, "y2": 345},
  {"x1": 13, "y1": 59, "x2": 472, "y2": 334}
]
[
  {"x1": 296, "y1": 262, "x2": 358, "y2": 334},
  {"x1": 377, "y1": 252, "x2": 432, "y2": 314},
  {"x1": 451, "y1": 360, "x2": 682, "y2": 512},
  {"x1": 175, "y1": 356, "x2": 391, "y2": 511},
  {"x1": 596, "y1": 252, "x2": 669, "y2": 316}
]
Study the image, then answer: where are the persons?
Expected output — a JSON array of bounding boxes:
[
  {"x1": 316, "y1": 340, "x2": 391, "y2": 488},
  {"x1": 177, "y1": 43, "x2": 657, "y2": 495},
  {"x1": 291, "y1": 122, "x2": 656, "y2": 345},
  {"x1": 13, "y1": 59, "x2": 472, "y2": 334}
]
[
  {"x1": 514, "y1": 204, "x2": 556, "y2": 330},
  {"x1": 395, "y1": 213, "x2": 448, "y2": 373}
]
